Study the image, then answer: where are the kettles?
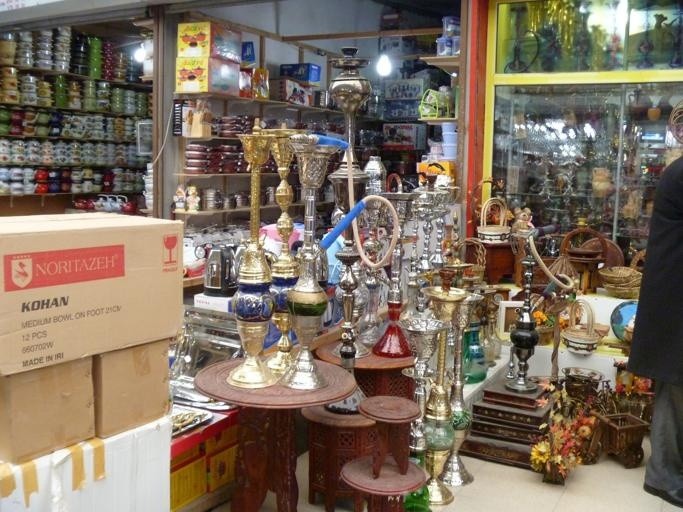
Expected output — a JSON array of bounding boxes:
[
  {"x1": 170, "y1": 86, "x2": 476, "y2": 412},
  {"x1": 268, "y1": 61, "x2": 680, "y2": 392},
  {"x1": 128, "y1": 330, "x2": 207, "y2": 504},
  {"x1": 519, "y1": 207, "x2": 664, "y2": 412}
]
[{"x1": 194, "y1": 240, "x2": 281, "y2": 297}]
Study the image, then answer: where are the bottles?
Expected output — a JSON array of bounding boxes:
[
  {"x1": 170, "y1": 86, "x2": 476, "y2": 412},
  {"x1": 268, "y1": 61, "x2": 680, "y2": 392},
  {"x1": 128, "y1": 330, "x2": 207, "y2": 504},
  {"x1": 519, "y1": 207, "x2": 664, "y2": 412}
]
[
  {"x1": 583, "y1": 101, "x2": 618, "y2": 135},
  {"x1": 499, "y1": 46, "x2": 531, "y2": 74},
  {"x1": 460, "y1": 309, "x2": 503, "y2": 385}
]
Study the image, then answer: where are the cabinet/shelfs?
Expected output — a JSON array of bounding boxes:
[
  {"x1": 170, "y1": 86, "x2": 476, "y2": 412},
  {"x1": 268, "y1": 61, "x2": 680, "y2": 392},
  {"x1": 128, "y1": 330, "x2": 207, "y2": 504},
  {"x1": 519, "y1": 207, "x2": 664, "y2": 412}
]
[{"x1": 467, "y1": 1, "x2": 681, "y2": 286}]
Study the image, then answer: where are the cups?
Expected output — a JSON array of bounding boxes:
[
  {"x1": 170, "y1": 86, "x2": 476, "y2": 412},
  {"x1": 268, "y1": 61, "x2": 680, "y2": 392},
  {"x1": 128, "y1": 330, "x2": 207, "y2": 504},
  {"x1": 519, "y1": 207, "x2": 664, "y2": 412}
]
[
  {"x1": 436, "y1": 15, "x2": 460, "y2": 58},
  {"x1": 420, "y1": 123, "x2": 457, "y2": 163}
]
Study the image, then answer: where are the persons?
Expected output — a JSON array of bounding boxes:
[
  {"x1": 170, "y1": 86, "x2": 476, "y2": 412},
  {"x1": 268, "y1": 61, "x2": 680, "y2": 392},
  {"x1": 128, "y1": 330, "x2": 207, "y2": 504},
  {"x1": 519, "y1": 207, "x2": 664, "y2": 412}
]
[{"x1": 626, "y1": 151, "x2": 682, "y2": 505}]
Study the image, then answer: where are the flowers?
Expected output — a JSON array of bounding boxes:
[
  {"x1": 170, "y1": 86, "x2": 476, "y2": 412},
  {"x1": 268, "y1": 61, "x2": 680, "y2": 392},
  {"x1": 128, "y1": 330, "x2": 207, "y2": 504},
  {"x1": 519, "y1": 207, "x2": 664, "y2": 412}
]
[{"x1": 530, "y1": 380, "x2": 592, "y2": 472}]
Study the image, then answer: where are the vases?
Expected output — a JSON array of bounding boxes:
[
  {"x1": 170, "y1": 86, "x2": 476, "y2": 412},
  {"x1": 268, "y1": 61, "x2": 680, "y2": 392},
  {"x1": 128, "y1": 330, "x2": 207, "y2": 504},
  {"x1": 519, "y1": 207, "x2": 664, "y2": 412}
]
[{"x1": 540, "y1": 460, "x2": 566, "y2": 485}]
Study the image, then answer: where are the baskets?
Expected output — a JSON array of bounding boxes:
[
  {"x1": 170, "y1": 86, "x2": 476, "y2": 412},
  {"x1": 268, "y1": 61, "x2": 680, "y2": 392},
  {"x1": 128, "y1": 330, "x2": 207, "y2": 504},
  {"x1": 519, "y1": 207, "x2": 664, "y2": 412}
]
[
  {"x1": 471, "y1": 180, "x2": 507, "y2": 224},
  {"x1": 477, "y1": 198, "x2": 511, "y2": 242},
  {"x1": 456, "y1": 238, "x2": 486, "y2": 284},
  {"x1": 560, "y1": 299, "x2": 610, "y2": 355},
  {"x1": 560, "y1": 228, "x2": 607, "y2": 272}
]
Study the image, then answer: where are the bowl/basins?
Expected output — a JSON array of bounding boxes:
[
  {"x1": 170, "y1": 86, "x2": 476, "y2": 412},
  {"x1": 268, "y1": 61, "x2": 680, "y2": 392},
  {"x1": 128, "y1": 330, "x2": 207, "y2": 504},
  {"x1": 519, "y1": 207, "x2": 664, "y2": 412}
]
[{"x1": 177, "y1": 113, "x2": 379, "y2": 212}]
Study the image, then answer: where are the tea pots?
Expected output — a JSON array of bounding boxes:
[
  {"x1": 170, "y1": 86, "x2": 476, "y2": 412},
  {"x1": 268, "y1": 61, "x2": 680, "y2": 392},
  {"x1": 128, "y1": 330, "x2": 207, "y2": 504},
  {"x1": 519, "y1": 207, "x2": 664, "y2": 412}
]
[
  {"x1": 1, "y1": 23, "x2": 154, "y2": 215},
  {"x1": 590, "y1": 167, "x2": 612, "y2": 197},
  {"x1": 626, "y1": 87, "x2": 653, "y2": 119}
]
